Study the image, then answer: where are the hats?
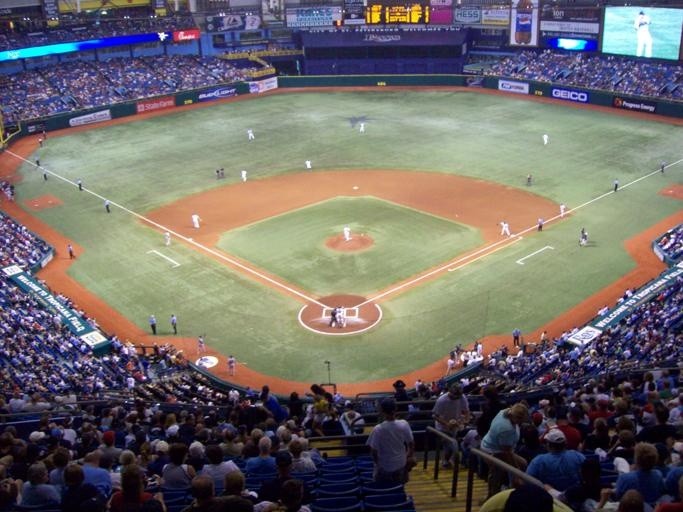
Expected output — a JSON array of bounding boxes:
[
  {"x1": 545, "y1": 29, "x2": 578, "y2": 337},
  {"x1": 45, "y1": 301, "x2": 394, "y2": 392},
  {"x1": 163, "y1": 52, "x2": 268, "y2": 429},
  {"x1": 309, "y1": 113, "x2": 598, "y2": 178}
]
[
  {"x1": 544, "y1": 429, "x2": 566, "y2": 443},
  {"x1": 29, "y1": 431, "x2": 45, "y2": 441}
]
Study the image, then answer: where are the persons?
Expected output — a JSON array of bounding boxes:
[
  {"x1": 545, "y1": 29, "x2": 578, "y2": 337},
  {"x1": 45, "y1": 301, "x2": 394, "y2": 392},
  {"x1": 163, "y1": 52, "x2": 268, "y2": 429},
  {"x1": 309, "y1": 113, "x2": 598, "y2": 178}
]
[
  {"x1": 35, "y1": 154, "x2": 40, "y2": 166},
  {"x1": 634, "y1": 10, "x2": 653, "y2": 56},
  {"x1": 660, "y1": 161, "x2": 666, "y2": 173},
  {"x1": 305, "y1": 160, "x2": 312, "y2": 170},
  {"x1": 614, "y1": 177, "x2": 618, "y2": 192},
  {"x1": 0, "y1": 178, "x2": 16, "y2": 202},
  {"x1": 104, "y1": 199, "x2": 110, "y2": 213},
  {"x1": 358, "y1": 121, "x2": 365, "y2": 134},
  {"x1": 466, "y1": 46, "x2": 683, "y2": 116},
  {"x1": 240, "y1": 168, "x2": 247, "y2": 182},
  {"x1": 215, "y1": 170, "x2": 219, "y2": 180},
  {"x1": 527, "y1": 173, "x2": 532, "y2": 186},
  {"x1": 0, "y1": 8, "x2": 304, "y2": 129},
  {"x1": 42, "y1": 170, "x2": 48, "y2": 180},
  {"x1": 247, "y1": 128, "x2": 255, "y2": 141},
  {"x1": 543, "y1": 133, "x2": 548, "y2": 146},
  {"x1": 0, "y1": 207, "x2": 682, "y2": 510},
  {"x1": 37, "y1": 137, "x2": 42, "y2": 148},
  {"x1": 219, "y1": 167, "x2": 224, "y2": 178},
  {"x1": 559, "y1": 202, "x2": 565, "y2": 219},
  {"x1": 41, "y1": 131, "x2": 46, "y2": 140},
  {"x1": 77, "y1": 178, "x2": 82, "y2": 191}
]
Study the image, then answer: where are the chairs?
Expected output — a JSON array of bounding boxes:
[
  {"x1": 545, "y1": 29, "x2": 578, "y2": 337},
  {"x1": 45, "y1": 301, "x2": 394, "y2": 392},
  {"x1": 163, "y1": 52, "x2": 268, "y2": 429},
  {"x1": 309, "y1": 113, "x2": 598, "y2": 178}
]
[{"x1": 0, "y1": 225, "x2": 683, "y2": 512}]
